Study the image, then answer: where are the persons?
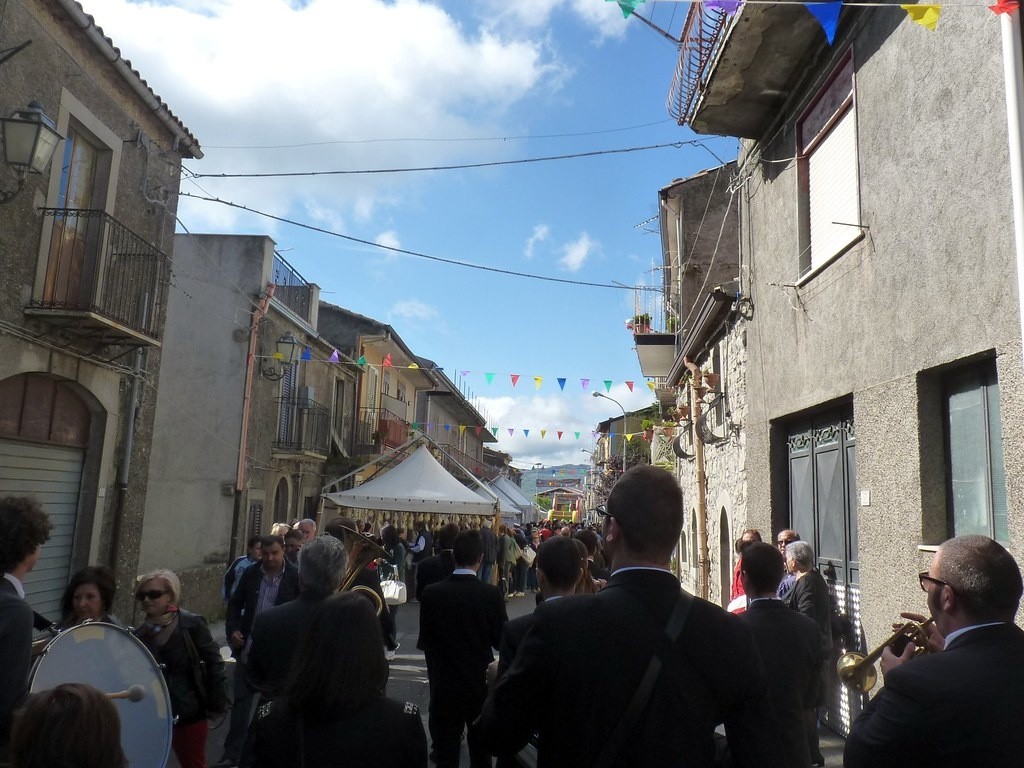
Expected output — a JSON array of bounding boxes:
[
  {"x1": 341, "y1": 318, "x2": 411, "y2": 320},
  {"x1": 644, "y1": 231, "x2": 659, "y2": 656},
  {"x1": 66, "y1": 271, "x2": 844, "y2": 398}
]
[
  {"x1": 842, "y1": 535, "x2": 1024, "y2": 768},
  {"x1": 0, "y1": 464, "x2": 830, "y2": 768}
]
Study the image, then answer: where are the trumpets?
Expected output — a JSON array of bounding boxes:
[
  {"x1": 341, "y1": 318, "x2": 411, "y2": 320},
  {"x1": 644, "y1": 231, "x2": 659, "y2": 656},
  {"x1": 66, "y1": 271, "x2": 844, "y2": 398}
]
[{"x1": 835, "y1": 616, "x2": 934, "y2": 695}]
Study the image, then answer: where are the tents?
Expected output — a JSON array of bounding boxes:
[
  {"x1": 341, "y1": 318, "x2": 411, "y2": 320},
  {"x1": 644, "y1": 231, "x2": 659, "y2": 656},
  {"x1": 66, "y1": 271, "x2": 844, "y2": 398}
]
[{"x1": 318, "y1": 445, "x2": 541, "y2": 531}]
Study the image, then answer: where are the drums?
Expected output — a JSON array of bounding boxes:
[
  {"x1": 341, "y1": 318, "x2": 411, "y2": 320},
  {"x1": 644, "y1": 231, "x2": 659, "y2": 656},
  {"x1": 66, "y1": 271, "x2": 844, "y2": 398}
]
[{"x1": 25, "y1": 618, "x2": 174, "y2": 768}]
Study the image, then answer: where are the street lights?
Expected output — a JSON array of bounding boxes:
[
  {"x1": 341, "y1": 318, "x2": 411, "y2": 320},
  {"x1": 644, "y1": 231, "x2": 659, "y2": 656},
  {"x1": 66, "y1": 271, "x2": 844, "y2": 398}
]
[
  {"x1": 580, "y1": 447, "x2": 596, "y2": 457},
  {"x1": 593, "y1": 391, "x2": 628, "y2": 474}
]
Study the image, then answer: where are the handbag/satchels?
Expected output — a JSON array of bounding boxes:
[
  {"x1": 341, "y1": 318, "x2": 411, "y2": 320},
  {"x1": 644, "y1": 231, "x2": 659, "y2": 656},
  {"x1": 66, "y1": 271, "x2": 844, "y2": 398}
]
[
  {"x1": 520, "y1": 545, "x2": 536, "y2": 565},
  {"x1": 181, "y1": 628, "x2": 227, "y2": 721},
  {"x1": 380, "y1": 565, "x2": 407, "y2": 605}
]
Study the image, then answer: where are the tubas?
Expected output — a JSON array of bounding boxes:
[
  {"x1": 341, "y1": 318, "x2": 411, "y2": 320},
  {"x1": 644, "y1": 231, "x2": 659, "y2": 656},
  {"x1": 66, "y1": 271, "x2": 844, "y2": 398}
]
[{"x1": 336, "y1": 522, "x2": 392, "y2": 620}]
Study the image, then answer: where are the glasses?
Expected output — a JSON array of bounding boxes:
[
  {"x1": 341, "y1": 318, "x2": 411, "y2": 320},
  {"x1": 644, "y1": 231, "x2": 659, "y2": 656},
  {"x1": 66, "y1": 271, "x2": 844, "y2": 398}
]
[
  {"x1": 136, "y1": 590, "x2": 169, "y2": 600},
  {"x1": 777, "y1": 541, "x2": 792, "y2": 545},
  {"x1": 919, "y1": 572, "x2": 954, "y2": 594},
  {"x1": 596, "y1": 505, "x2": 614, "y2": 517},
  {"x1": 534, "y1": 537, "x2": 539, "y2": 539}
]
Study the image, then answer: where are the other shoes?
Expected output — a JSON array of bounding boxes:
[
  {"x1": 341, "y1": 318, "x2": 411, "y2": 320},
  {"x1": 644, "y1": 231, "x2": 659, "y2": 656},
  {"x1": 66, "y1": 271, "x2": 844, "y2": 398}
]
[
  {"x1": 532, "y1": 587, "x2": 541, "y2": 593},
  {"x1": 507, "y1": 591, "x2": 524, "y2": 597},
  {"x1": 383, "y1": 643, "x2": 400, "y2": 660}
]
